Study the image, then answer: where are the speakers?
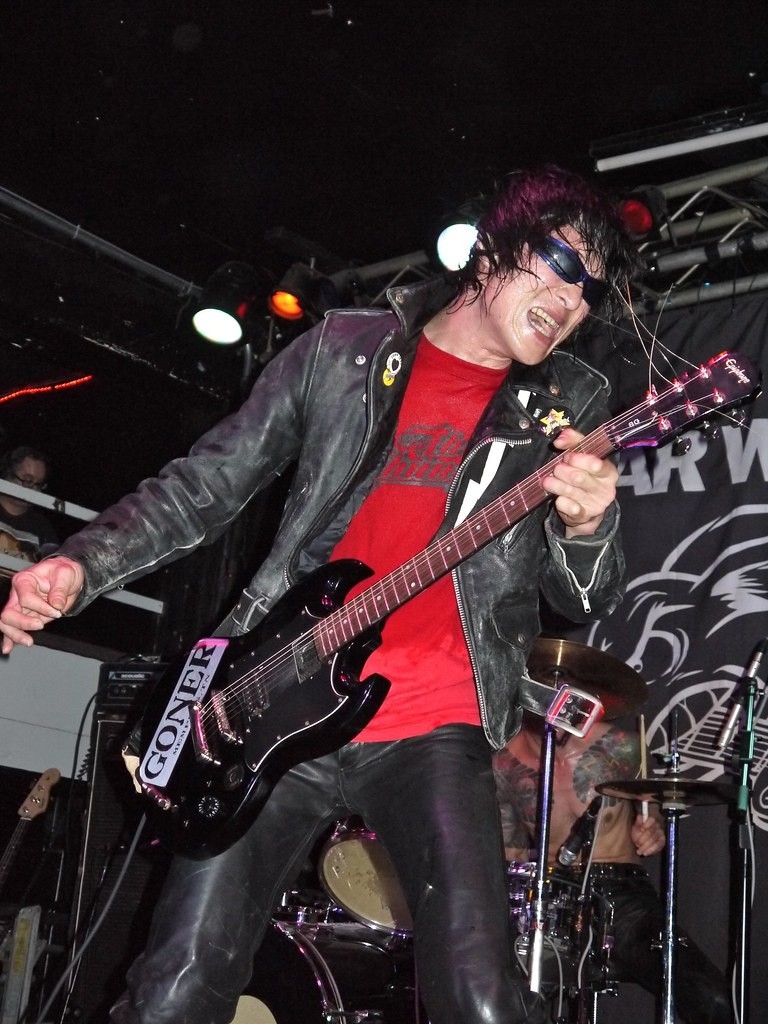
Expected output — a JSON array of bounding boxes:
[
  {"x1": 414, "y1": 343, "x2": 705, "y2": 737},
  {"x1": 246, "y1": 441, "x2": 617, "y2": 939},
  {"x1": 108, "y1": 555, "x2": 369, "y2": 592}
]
[{"x1": 55, "y1": 711, "x2": 166, "y2": 1024}]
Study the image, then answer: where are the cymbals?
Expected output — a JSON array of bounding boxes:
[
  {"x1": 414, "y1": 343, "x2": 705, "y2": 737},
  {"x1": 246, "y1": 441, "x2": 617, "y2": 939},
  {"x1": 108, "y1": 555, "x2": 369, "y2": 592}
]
[
  {"x1": 594, "y1": 775, "x2": 737, "y2": 806},
  {"x1": 518, "y1": 636, "x2": 649, "y2": 721}
]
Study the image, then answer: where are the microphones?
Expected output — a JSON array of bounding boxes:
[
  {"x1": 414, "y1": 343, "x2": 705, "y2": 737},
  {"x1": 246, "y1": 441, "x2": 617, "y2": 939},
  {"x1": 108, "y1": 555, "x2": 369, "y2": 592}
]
[
  {"x1": 558, "y1": 796, "x2": 604, "y2": 866},
  {"x1": 718, "y1": 639, "x2": 768, "y2": 747}
]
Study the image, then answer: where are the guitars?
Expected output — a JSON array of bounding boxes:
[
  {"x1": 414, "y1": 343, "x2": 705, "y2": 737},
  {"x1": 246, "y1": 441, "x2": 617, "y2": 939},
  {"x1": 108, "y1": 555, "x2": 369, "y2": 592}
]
[
  {"x1": 0, "y1": 765, "x2": 62, "y2": 886},
  {"x1": 136, "y1": 345, "x2": 766, "y2": 861}
]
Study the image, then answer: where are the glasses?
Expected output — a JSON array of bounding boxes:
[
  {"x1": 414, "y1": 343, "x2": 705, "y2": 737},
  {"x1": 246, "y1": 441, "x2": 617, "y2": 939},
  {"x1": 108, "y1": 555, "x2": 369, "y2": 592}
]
[{"x1": 526, "y1": 229, "x2": 609, "y2": 307}]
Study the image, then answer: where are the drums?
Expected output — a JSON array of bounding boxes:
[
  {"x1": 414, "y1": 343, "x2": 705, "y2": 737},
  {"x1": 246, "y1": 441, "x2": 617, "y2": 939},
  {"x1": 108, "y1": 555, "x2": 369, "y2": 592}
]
[
  {"x1": 317, "y1": 828, "x2": 416, "y2": 954},
  {"x1": 505, "y1": 861, "x2": 615, "y2": 971},
  {"x1": 231, "y1": 916, "x2": 432, "y2": 1023}
]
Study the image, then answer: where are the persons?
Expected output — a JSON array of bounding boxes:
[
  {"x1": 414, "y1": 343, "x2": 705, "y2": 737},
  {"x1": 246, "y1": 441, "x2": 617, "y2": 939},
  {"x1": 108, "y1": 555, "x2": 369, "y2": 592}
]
[
  {"x1": 495, "y1": 719, "x2": 742, "y2": 1024},
  {"x1": 0, "y1": 437, "x2": 61, "y2": 561},
  {"x1": 0, "y1": 164, "x2": 642, "y2": 1023}
]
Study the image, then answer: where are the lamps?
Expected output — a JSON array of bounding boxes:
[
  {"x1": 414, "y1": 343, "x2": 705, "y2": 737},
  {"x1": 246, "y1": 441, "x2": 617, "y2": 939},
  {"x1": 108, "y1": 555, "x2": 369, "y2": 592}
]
[
  {"x1": 192, "y1": 283, "x2": 252, "y2": 346},
  {"x1": 432, "y1": 200, "x2": 491, "y2": 274},
  {"x1": 268, "y1": 263, "x2": 320, "y2": 324}
]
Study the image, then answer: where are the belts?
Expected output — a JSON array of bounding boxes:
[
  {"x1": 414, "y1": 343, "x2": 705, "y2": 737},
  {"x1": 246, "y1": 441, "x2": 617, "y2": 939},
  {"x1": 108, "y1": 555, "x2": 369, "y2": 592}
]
[{"x1": 572, "y1": 862, "x2": 651, "y2": 880}]
